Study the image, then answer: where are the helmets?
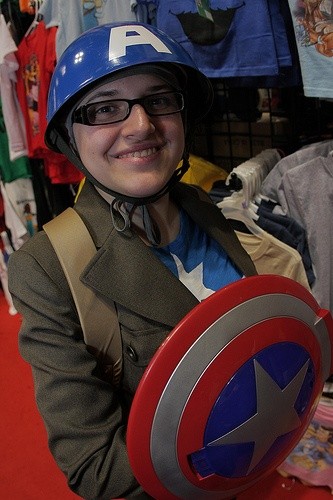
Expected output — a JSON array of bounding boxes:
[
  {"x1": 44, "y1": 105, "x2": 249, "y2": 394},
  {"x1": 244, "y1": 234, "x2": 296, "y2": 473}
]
[{"x1": 44, "y1": 21, "x2": 215, "y2": 152}]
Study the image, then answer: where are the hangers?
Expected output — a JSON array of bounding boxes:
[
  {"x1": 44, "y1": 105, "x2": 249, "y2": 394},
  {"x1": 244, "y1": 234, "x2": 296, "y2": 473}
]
[
  {"x1": 215, "y1": 148, "x2": 282, "y2": 240},
  {"x1": 23, "y1": 0, "x2": 45, "y2": 38}
]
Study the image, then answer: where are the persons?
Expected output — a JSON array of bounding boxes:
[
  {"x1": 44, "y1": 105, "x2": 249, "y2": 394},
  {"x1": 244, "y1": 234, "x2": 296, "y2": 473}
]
[{"x1": 5, "y1": 21, "x2": 259, "y2": 500}]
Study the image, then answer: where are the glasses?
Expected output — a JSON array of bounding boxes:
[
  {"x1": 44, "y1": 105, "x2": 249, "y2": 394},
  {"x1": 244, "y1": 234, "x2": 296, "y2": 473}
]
[{"x1": 72, "y1": 91, "x2": 186, "y2": 126}]
